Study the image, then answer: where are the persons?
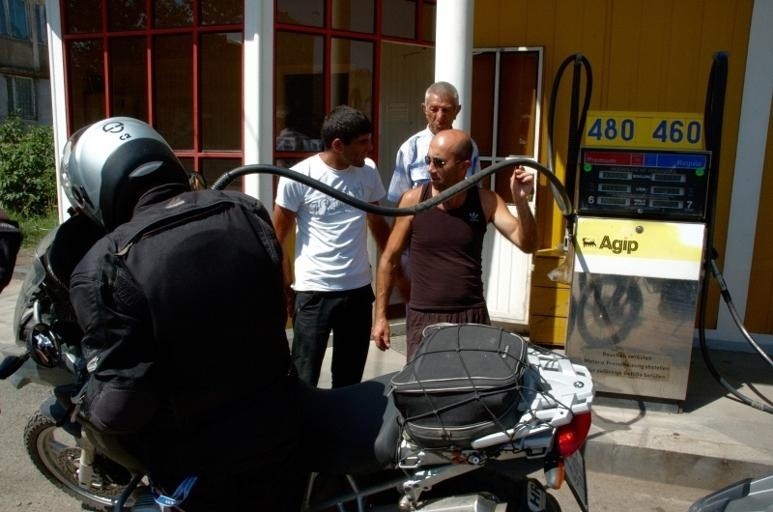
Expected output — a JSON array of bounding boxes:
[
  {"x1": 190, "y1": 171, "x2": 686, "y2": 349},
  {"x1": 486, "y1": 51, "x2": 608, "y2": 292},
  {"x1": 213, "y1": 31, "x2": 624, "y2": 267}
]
[
  {"x1": 370, "y1": 128, "x2": 542, "y2": 364},
  {"x1": 59, "y1": 114, "x2": 314, "y2": 512},
  {"x1": 270, "y1": 104, "x2": 412, "y2": 390},
  {"x1": 386, "y1": 80, "x2": 483, "y2": 278}
]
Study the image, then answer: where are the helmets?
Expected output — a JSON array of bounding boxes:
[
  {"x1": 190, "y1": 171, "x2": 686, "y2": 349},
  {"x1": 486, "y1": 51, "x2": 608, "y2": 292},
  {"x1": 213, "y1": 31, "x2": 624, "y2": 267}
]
[{"x1": 58, "y1": 115, "x2": 193, "y2": 232}]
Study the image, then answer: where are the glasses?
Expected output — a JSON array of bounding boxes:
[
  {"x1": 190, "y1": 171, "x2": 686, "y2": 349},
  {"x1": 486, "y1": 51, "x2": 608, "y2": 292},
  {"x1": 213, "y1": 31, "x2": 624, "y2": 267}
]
[{"x1": 424, "y1": 155, "x2": 447, "y2": 169}]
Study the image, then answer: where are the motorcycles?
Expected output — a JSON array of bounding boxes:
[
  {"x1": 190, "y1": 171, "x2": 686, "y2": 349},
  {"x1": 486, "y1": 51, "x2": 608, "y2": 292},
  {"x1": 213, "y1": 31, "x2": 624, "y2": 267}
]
[{"x1": 0, "y1": 211, "x2": 597, "y2": 511}]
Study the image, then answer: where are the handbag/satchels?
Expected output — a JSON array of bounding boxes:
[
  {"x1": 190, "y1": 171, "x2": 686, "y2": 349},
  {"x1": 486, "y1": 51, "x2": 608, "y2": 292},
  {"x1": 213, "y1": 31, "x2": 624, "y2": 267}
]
[{"x1": 389, "y1": 326, "x2": 529, "y2": 448}]
